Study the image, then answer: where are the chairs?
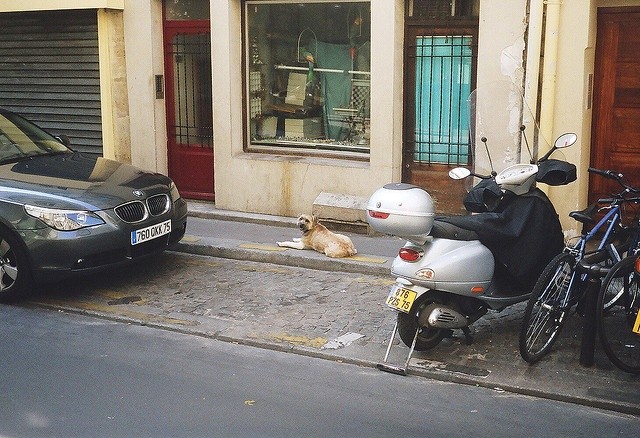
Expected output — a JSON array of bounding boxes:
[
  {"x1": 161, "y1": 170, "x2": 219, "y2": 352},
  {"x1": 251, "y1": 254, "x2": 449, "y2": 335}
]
[{"x1": 332, "y1": 100, "x2": 366, "y2": 140}]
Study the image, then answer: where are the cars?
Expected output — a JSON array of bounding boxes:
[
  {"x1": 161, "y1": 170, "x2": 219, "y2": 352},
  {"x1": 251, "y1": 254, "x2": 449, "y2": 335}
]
[{"x1": 0, "y1": 108, "x2": 189, "y2": 302}]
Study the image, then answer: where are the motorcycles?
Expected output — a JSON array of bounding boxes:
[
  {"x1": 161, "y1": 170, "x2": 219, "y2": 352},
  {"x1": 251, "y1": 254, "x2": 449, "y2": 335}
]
[{"x1": 365, "y1": 130, "x2": 577, "y2": 353}]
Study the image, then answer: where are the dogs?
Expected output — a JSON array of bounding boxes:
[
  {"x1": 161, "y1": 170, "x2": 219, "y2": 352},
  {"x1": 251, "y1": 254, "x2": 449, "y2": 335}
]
[{"x1": 276, "y1": 213, "x2": 357, "y2": 258}]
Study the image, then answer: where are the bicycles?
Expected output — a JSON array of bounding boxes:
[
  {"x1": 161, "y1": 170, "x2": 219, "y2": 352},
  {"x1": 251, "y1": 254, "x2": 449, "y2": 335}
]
[
  {"x1": 519, "y1": 165, "x2": 640, "y2": 365},
  {"x1": 596, "y1": 252, "x2": 639, "y2": 374}
]
